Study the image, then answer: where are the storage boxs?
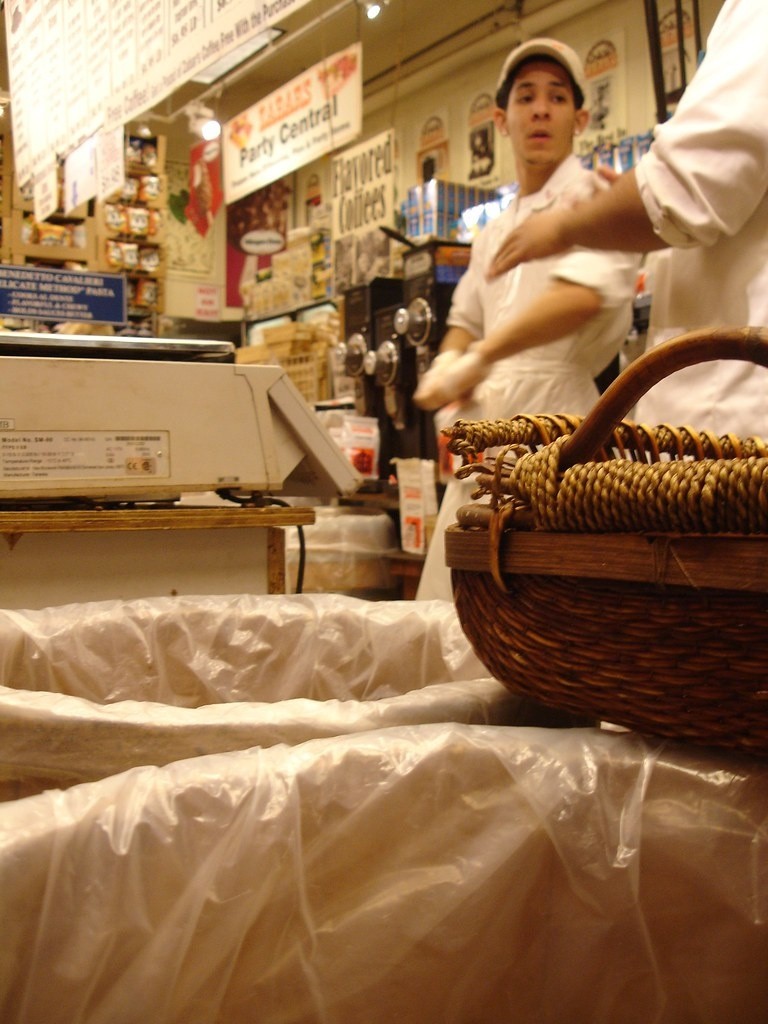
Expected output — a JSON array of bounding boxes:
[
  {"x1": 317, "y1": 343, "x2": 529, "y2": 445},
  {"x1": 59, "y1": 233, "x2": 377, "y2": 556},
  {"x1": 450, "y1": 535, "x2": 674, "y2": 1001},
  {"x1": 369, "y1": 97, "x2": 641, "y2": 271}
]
[
  {"x1": 96, "y1": 234, "x2": 168, "y2": 280},
  {"x1": 94, "y1": 202, "x2": 168, "y2": 245},
  {"x1": 11, "y1": 208, "x2": 97, "y2": 262},
  {"x1": 404, "y1": 135, "x2": 652, "y2": 240},
  {"x1": 124, "y1": 130, "x2": 168, "y2": 174},
  {"x1": 137, "y1": 279, "x2": 167, "y2": 313},
  {"x1": 104, "y1": 173, "x2": 168, "y2": 208},
  {"x1": 12, "y1": 172, "x2": 88, "y2": 221}
]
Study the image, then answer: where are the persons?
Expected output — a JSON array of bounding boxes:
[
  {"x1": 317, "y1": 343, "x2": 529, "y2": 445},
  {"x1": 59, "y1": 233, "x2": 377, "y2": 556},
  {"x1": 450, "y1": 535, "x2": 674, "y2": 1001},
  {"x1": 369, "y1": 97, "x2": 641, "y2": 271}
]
[
  {"x1": 414, "y1": 37, "x2": 637, "y2": 599},
  {"x1": 485, "y1": 0, "x2": 768, "y2": 466}
]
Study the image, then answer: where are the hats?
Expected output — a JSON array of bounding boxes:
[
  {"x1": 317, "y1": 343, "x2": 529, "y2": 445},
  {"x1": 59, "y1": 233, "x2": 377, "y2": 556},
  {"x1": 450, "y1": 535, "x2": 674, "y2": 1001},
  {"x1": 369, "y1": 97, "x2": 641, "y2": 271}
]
[{"x1": 497, "y1": 38, "x2": 592, "y2": 110}]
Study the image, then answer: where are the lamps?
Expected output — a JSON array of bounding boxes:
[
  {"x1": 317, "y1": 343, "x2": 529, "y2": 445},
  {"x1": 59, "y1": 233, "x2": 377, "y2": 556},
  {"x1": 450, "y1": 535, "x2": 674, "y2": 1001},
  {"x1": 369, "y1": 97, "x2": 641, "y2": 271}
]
[
  {"x1": 356, "y1": 0, "x2": 381, "y2": 20},
  {"x1": 184, "y1": 105, "x2": 222, "y2": 140}
]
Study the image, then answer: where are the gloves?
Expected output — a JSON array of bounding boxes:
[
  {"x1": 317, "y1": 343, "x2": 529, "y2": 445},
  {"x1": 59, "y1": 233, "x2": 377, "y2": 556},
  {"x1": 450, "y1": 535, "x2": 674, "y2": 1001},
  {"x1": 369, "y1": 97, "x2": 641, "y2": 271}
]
[{"x1": 413, "y1": 350, "x2": 490, "y2": 410}]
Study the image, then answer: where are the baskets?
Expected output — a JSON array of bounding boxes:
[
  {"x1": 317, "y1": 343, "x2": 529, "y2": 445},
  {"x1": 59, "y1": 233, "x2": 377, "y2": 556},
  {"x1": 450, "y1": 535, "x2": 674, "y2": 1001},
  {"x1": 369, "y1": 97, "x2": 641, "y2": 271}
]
[{"x1": 444, "y1": 324, "x2": 768, "y2": 755}]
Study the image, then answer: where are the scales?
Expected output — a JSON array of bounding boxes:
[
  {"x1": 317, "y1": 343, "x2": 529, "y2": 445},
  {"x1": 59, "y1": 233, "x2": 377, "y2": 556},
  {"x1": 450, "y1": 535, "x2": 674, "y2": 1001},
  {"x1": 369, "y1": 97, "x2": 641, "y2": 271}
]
[{"x1": 0, "y1": 333, "x2": 365, "y2": 504}]
